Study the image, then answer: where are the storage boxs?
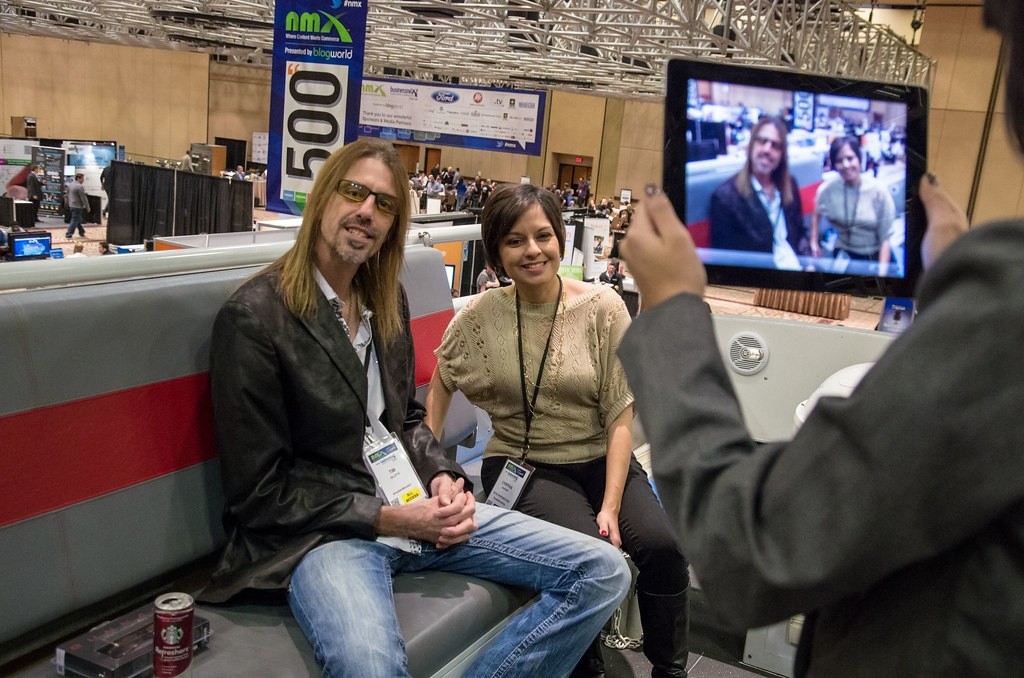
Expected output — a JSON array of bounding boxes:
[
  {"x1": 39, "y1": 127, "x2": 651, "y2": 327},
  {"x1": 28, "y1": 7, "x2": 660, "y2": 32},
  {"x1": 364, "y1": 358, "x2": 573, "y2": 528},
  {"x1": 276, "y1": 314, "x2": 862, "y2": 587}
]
[{"x1": 50, "y1": 600, "x2": 214, "y2": 678}]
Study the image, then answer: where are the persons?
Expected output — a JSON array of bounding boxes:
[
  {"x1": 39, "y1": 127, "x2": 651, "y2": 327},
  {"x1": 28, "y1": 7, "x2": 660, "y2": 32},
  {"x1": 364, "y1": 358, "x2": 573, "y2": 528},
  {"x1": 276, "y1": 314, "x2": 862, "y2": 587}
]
[
  {"x1": 708, "y1": 101, "x2": 906, "y2": 278},
  {"x1": 65, "y1": 242, "x2": 116, "y2": 258},
  {"x1": 406, "y1": 163, "x2": 497, "y2": 211},
  {"x1": 196, "y1": 136, "x2": 631, "y2": 678},
  {"x1": 614, "y1": 0, "x2": 1024, "y2": 677},
  {"x1": 27, "y1": 165, "x2": 46, "y2": 223},
  {"x1": 594, "y1": 236, "x2": 603, "y2": 254},
  {"x1": 66, "y1": 173, "x2": 90, "y2": 238},
  {"x1": 545, "y1": 174, "x2": 629, "y2": 233},
  {"x1": 599, "y1": 263, "x2": 623, "y2": 295},
  {"x1": 100, "y1": 164, "x2": 111, "y2": 219},
  {"x1": 232, "y1": 165, "x2": 246, "y2": 180},
  {"x1": 424, "y1": 183, "x2": 691, "y2": 678},
  {"x1": 181, "y1": 150, "x2": 194, "y2": 173},
  {"x1": 477, "y1": 263, "x2": 500, "y2": 294}
]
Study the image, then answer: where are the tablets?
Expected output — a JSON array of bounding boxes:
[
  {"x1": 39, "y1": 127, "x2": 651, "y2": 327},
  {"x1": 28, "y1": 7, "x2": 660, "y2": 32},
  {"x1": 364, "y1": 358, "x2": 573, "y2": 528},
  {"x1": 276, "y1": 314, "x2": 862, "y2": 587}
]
[{"x1": 660, "y1": 58, "x2": 927, "y2": 298}]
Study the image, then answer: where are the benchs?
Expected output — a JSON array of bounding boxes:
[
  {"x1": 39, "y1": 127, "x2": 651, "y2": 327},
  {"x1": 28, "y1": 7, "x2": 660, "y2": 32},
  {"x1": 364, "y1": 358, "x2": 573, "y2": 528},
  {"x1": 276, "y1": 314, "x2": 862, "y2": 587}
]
[
  {"x1": 0, "y1": 244, "x2": 544, "y2": 678},
  {"x1": 687, "y1": 154, "x2": 832, "y2": 251}
]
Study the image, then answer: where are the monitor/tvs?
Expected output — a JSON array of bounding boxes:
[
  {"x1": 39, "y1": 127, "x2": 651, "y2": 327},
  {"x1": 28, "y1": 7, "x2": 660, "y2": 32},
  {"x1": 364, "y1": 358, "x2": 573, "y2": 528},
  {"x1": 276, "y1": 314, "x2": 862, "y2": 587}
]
[{"x1": 8, "y1": 231, "x2": 52, "y2": 261}]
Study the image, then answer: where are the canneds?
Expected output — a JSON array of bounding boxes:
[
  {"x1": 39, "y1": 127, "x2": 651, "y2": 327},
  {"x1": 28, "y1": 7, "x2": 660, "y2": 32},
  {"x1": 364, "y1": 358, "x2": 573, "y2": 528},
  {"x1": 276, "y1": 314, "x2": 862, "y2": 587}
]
[{"x1": 153, "y1": 592, "x2": 194, "y2": 678}]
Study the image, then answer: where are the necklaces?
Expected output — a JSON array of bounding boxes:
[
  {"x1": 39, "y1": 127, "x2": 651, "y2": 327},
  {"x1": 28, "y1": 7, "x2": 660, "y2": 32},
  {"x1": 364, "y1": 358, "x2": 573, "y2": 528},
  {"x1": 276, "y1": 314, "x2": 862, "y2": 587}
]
[{"x1": 345, "y1": 288, "x2": 353, "y2": 325}]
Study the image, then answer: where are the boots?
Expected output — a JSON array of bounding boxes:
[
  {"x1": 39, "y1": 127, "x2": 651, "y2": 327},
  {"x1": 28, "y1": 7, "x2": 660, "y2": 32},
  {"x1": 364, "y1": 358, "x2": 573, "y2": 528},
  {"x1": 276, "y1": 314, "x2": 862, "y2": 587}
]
[
  {"x1": 633, "y1": 571, "x2": 690, "y2": 678},
  {"x1": 571, "y1": 633, "x2": 605, "y2": 678}
]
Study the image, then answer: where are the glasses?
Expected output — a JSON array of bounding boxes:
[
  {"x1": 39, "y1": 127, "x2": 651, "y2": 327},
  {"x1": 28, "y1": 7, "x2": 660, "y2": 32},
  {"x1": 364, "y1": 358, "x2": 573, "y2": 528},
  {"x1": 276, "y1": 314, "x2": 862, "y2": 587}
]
[
  {"x1": 336, "y1": 179, "x2": 403, "y2": 216},
  {"x1": 755, "y1": 134, "x2": 783, "y2": 152}
]
